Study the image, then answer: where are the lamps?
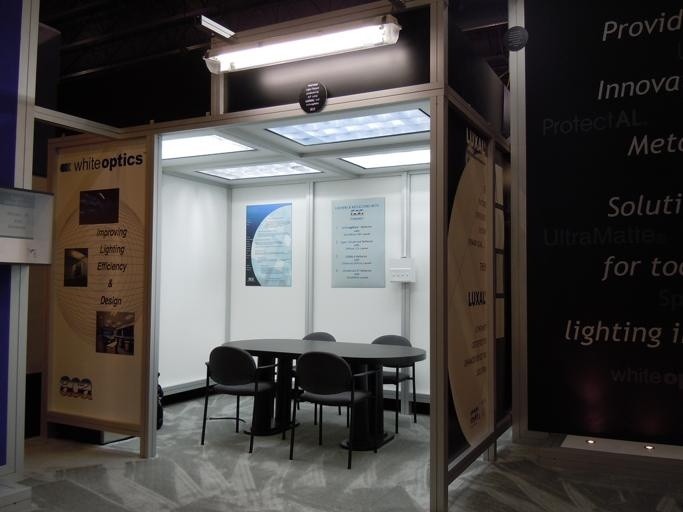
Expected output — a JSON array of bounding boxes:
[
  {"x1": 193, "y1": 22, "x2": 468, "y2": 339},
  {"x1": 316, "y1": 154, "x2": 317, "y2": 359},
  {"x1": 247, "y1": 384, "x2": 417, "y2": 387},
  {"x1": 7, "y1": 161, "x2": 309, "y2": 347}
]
[{"x1": 203, "y1": 14, "x2": 401, "y2": 74}]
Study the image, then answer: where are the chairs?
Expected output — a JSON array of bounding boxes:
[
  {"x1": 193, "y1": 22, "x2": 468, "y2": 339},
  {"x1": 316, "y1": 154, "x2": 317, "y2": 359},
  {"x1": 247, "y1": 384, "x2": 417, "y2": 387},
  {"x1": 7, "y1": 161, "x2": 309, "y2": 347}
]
[
  {"x1": 289, "y1": 351, "x2": 380, "y2": 469},
  {"x1": 371, "y1": 335, "x2": 417, "y2": 433},
  {"x1": 201, "y1": 346, "x2": 287, "y2": 452},
  {"x1": 294, "y1": 333, "x2": 341, "y2": 425}
]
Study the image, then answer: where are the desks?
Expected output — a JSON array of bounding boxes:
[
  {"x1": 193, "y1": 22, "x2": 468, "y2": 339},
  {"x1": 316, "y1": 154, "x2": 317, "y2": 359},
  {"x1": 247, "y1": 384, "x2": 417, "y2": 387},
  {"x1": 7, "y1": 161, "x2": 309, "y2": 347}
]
[{"x1": 223, "y1": 338, "x2": 426, "y2": 451}]
